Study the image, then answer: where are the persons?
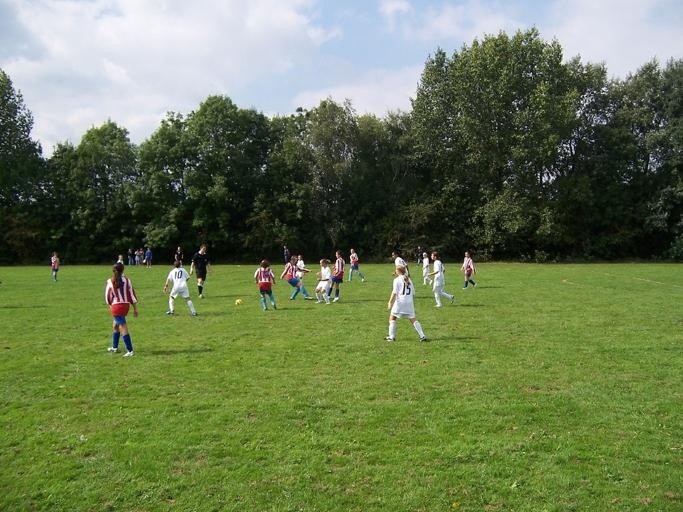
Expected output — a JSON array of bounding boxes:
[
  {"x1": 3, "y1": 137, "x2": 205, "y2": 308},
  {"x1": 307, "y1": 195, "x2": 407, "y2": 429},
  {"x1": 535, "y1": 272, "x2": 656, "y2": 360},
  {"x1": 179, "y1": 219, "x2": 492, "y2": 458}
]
[
  {"x1": 163, "y1": 261, "x2": 196, "y2": 315},
  {"x1": 189, "y1": 243, "x2": 208, "y2": 298},
  {"x1": 104, "y1": 262, "x2": 138, "y2": 358},
  {"x1": 425, "y1": 249, "x2": 455, "y2": 308},
  {"x1": 460, "y1": 252, "x2": 477, "y2": 289},
  {"x1": 50, "y1": 251, "x2": 58, "y2": 282},
  {"x1": 253, "y1": 260, "x2": 276, "y2": 311},
  {"x1": 346, "y1": 248, "x2": 366, "y2": 282},
  {"x1": 283, "y1": 246, "x2": 290, "y2": 264},
  {"x1": 416, "y1": 246, "x2": 423, "y2": 265},
  {"x1": 280, "y1": 251, "x2": 345, "y2": 306},
  {"x1": 174, "y1": 246, "x2": 183, "y2": 267},
  {"x1": 390, "y1": 250, "x2": 409, "y2": 281},
  {"x1": 116, "y1": 253, "x2": 124, "y2": 265},
  {"x1": 383, "y1": 265, "x2": 427, "y2": 342},
  {"x1": 422, "y1": 252, "x2": 433, "y2": 286},
  {"x1": 126, "y1": 247, "x2": 152, "y2": 269}
]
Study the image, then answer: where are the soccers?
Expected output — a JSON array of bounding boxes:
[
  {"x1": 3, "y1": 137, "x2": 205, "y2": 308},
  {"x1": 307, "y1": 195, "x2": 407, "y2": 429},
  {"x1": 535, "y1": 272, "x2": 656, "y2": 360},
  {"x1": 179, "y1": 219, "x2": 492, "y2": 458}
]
[{"x1": 235, "y1": 298, "x2": 244, "y2": 306}]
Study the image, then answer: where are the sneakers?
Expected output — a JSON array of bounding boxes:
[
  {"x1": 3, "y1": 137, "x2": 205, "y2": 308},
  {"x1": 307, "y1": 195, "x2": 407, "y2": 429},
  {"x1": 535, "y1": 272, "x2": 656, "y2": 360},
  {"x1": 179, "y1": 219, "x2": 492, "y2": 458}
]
[
  {"x1": 419, "y1": 336, "x2": 428, "y2": 342},
  {"x1": 198, "y1": 293, "x2": 204, "y2": 299},
  {"x1": 383, "y1": 335, "x2": 395, "y2": 342},
  {"x1": 472, "y1": 283, "x2": 476, "y2": 289},
  {"x1": 315, "y1": 297, "x2": 339, "y2": 305},
  {"x1": 273, "y1": 304, "x2": 277, "y2": 310},
  {"x1": 305, "y1": 296, "x2": 313, "y2": 300},
  {"x1": 192, "y1": 312, "x2": 197, "y2": 316},
  {"x1": 462, "y1": 286, "x2": 468, "y2": 290},
  {"x1": 450, "y1": 295, "x2": 454, "y2": 304},
  {"x1": 422, "y1": 280, "x2": 434, "y2": 286},
  {"x1": 290, "y1": 297, "x2": 295, "y2": 300},
  {"x1": 107, "y1": 347, "x2": 119, "y2": 353},
  {"x1": 122, "y1": 351, "x2": 134, "y2": 357},
  {"x1": 347, "y1": 277, "x2": 367, "y2": 282},
  {"x1": 263, "y1": 306, "x2": 268, "y2": 310},
  {"x1": 166, "y1": 311, "x2": 174, "y2": 315},
  {"x1": 432, "y1": 303, "x2": 442, "y2": 308}
]
